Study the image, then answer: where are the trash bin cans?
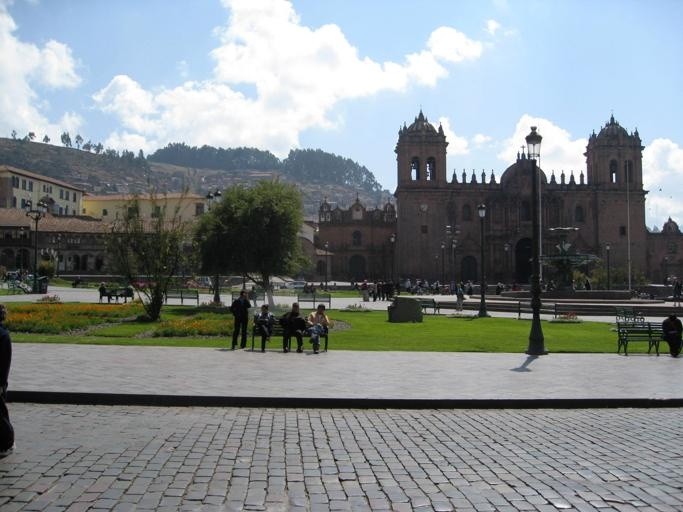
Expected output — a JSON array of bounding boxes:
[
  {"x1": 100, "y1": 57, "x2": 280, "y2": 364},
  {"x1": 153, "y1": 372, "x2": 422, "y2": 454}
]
[{"x1": 37, "y1": 276, "x2": 47, "y2": 294}]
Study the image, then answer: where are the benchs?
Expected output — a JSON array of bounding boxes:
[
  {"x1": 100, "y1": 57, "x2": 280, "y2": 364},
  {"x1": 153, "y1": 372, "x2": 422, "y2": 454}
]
[
  {"x1": 416, "y1": 298, "x2": 440, "y2": 314},
  {"x1": 252, "y1": 315, "x2": 328, "y2": 352},
  {"x1": 166, "y1": 289, "x2": 199, "y2": 305},
  {"x1": 298, "y1": 293, "x2": 331, "y2": 310},
  {"x1": 232, "y1": 290, "x2": 265, "y2": 306},
  {"x1": 617, "y1": 322, "x2": 683, "y2": 356},
  {"x1": 518, "y1": 301, "x2": 557, "y2": 318},
  {"x1": 100, "y1": 288, "x2": 134, "y2": 303}
]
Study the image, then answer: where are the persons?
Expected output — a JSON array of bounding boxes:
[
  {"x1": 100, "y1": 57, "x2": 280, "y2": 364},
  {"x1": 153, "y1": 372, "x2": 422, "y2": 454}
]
[
  {"x1": 98, "y1": 282, "x2": 114, "y2": 301},
  {"x1": 307, "y1": 304, "x2": 329, "y2": 354},
  {"x1": 124, "y1": 282, "x2": 134, "y2": 303},
  {"x1": 230, "y1": 290, "x2": 252, "y2": 349},
  {"x1": 253, "y1": 304, "x2": 276, "y2": 352},
  {"x1": 496, "y1": 278, "x2": 592, "y2": 296},
  {"x1": 302, "y1": 278, "x2": 474, "y2": 312},
  {"x1": 278, "y1": 302, "x2": 306, "y2": 353},
  {"x1": 249, "y1": 287, "x2": 257, "y2": 300},
  {"x1": 0, "y1": 304, "x2": 16, "y2": 456},
  {"x1": 661, "y1": 312, "x2": 683, "y2": 357},
  {"x1": 1, "y1": 272, "x2": 32, "y2": 293},
  {"x1": 632, "y1": 272, "x2": 683, "y2": 306},
  {"x1": 72, "y1": 276, "x2": 82, "y2": 288}
]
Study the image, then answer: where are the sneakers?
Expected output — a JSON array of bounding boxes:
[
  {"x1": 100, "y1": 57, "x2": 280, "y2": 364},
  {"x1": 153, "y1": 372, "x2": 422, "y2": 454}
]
[
  {"x1": 0, "y1": 441, "x2": 16, "y2": 457},
  {"x1": 309, "y1": 336, "x2": 315, "y2": 343},
  {"x1": 231, "y1": 344, "x2": 319, "y2": 354}
]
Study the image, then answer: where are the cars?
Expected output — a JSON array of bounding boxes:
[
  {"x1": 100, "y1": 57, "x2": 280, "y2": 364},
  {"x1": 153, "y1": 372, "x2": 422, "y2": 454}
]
[
  {"x1": 180, "y1": 273, "x2": 306, "y2": 293},
  {"x1": 128, "y1": 278, "x2": 159, "y2": 289},
  {"x1": 0, "y1": 265, "x2": 34, "y2": 284}
]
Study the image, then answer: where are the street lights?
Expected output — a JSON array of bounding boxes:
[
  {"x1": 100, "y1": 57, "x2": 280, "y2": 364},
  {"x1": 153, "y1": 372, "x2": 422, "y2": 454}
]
[
  {"x1": 476, "y1": 202, "x2": 489, "y2": 316},
  {"x1": 23, "y1": 201, "x2": 49, "y2": 292},
  {"x1": 53, "y1": 233, "x2": 63, "y2": 276},
  {"x1": 435, "y1": 224, "x2": 460, "y2": 287},
  {"x1": 503, "y1": 242, "x2": 511, "y2": 273},
  {"x1": 604, "y1": 242, "x2": 612, "y2": 290},
  {"x1": 324, "y1": 240, "x2": 328, "y2": 292},
  {"x1": 389, "y1": 233, "x2": 396, "y2": 281},
  {"x1": 663, "y1": 257, "x2": 669, "y2": 276},
  {"x1": 523, "y1": 125, "x2": 549, "y2": 353}
]
